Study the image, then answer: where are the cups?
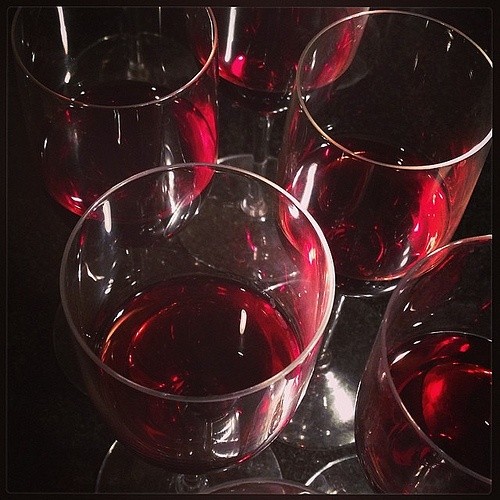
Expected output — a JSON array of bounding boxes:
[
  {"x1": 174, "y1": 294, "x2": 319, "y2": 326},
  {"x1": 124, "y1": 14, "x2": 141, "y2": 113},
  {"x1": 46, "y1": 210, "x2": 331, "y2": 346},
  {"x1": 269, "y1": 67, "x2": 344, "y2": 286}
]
[{"x1": 8, "y1": 2, "x2": 496, "y2": 495}]
